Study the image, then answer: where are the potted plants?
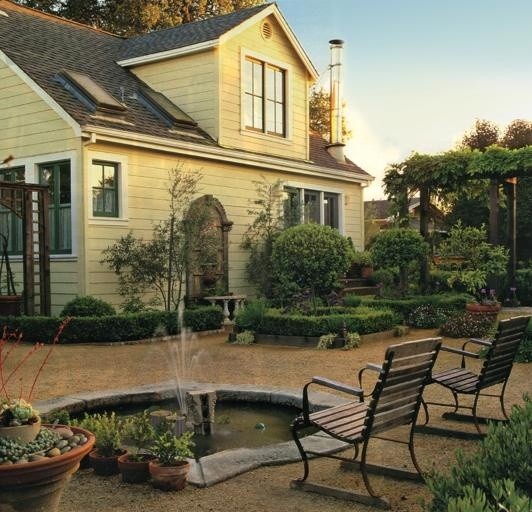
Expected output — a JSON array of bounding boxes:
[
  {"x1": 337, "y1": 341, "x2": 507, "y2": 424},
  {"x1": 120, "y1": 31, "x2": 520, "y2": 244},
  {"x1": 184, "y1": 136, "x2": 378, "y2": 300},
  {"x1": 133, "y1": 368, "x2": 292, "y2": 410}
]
[
  {"x1": 1, "y1": 396, "x2": 197, "y2": 512},
  {"x1": 354, "y1": 250, "x2": 374, "y2": 279}
]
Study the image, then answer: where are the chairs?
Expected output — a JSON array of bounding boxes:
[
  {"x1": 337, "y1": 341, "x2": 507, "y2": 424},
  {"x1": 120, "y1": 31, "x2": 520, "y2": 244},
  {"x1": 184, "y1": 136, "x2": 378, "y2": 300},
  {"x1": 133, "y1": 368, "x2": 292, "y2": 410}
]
[
  {"x1": 421, "y1": 313, "x2": 529, "y2": 442},
  {"x1": 291, "y1": 333, "x2": 448, "y2": 508}
]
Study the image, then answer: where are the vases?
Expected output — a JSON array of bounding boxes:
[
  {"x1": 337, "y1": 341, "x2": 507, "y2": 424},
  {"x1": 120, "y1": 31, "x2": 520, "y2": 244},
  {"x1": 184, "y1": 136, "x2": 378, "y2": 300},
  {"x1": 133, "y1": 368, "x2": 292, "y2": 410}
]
[{"x1": 465, "y1": 303, "x2": 500, "y2": 322}]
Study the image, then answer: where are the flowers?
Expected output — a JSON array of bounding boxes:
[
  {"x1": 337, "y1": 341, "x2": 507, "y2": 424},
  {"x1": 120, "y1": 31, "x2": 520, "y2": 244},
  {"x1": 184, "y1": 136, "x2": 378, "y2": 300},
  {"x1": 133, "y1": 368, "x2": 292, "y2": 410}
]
[{"x1": 469, "y1": 288, "x2": 498, "y2": 305}]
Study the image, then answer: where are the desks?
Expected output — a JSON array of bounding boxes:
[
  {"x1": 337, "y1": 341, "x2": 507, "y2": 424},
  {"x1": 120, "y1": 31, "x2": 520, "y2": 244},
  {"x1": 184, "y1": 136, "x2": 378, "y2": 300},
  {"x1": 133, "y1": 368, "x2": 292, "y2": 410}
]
[{"x1": 204, "y1": 294, "x2": 247, "y2": 328}]
